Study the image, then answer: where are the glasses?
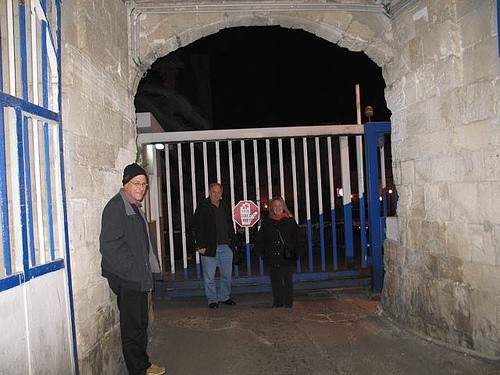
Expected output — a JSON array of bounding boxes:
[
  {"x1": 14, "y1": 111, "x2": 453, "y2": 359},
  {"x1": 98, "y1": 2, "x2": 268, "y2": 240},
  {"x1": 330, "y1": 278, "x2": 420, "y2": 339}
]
[{"x1": 130, "y1": 181, "x2": 148, "y2": 187}]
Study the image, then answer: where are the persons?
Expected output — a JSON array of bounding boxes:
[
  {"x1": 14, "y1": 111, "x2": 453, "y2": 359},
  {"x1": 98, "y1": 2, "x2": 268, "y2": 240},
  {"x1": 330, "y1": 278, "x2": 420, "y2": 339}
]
[
  {"x1": 190, "y1": 183, "x2": 240, "y2": 308},
  {"x1": 256, "y1": 196, "x2": 302, "y2": 308},
  {"x1": 99, "y1": 163, "x2": 166, "y2": 374}
]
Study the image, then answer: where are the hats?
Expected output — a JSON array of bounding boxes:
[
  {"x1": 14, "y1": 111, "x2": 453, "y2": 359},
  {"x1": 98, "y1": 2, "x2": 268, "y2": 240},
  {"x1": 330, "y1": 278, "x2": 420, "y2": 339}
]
[{"x1": 121, "y1": 162, "x2": 147, "y2": 186}]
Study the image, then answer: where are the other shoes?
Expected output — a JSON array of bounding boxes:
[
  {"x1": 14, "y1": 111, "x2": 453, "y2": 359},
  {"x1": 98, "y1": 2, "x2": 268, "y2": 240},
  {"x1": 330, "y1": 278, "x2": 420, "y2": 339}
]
[
  {"x1": 220, "y1": 299, "x2": 235, "y2": 305},
  {"x1": 272, "y1": 303, "x2": 283, "y2": 308},
  {"x1": 285, "y1": 303, "x2": 293, "y2": 309},
  {"x1": 147, "y1": 364, "x2": 166, "y2": 375},
  {"x1": 209, "y1": 302, "x2": 218, "y2": 308}
]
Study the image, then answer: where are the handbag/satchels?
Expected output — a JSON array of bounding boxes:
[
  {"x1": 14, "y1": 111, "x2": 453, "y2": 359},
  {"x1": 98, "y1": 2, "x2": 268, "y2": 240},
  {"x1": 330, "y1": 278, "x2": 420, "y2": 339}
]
[{"x1": 283, "y1": 246, "x2": 298, "y2": 261}]
